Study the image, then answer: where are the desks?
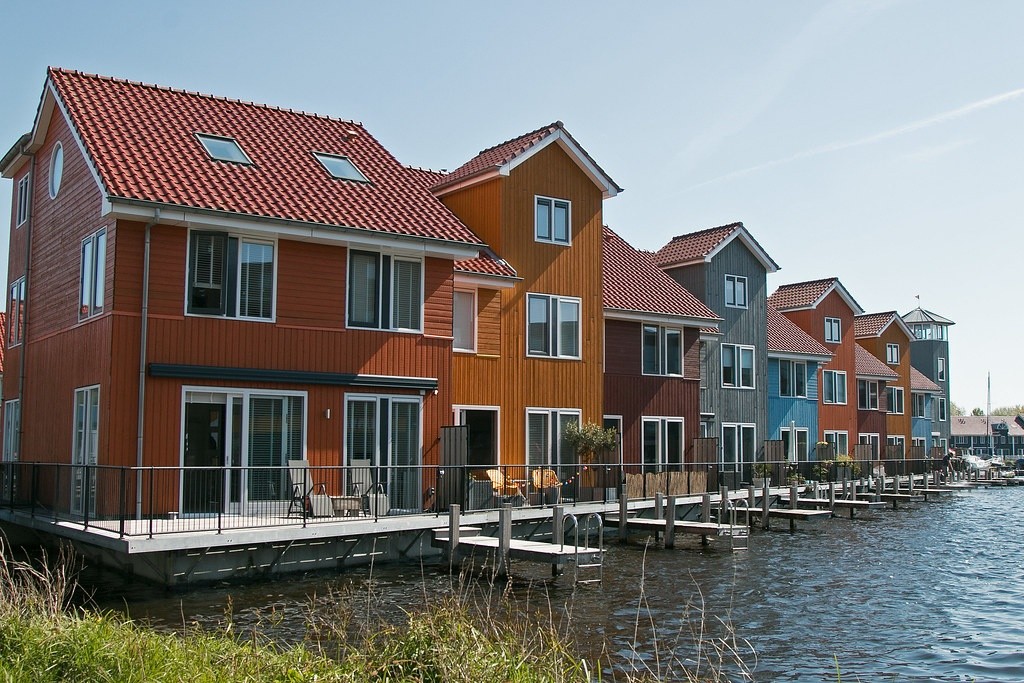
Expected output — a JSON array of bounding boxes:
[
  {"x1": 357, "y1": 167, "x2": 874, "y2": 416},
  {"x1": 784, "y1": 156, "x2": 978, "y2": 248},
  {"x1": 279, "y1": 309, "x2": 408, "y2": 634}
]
[
  {"x1": 329, "y1": 496, "x2": 361, "y2": 517},
  {"x1": 513, "y1": 479, "x2": 528, "y2": 492}
]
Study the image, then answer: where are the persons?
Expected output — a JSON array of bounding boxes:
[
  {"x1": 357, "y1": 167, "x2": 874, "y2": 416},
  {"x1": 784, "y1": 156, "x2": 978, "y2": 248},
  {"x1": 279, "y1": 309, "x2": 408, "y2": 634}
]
[{"x1": 942, "y1": 452, "x2": 953, "y2": 482}]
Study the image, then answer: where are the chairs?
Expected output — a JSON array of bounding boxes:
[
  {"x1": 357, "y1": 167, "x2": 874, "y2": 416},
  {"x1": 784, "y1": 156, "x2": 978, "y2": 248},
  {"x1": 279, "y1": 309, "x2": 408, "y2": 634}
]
[
  {"x1": 287, "y1": 460, "x2": 336, "y2": 518},
  {"x1": 350, "y1": 459, "x2": 390, "y2": 517},
  {"x1": 532, "y1": 469, "x2": 563, "y2": 494},
  {"x1": 486, "y1": 469, "x2": 517, "y2": 497}
]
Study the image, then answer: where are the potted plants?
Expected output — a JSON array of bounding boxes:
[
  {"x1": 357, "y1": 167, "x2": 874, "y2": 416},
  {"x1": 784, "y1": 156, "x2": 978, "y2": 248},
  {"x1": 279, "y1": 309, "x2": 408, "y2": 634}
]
[{"x1": 752, "y1": 441, "x2": 861, "y2": 488}]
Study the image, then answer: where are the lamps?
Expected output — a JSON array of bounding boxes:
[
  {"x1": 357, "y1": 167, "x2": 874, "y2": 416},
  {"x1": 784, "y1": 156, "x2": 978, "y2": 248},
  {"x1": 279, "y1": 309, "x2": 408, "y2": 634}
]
[{"x1": 325, "y1": 409, "x2": 331, "y2": 419}]
